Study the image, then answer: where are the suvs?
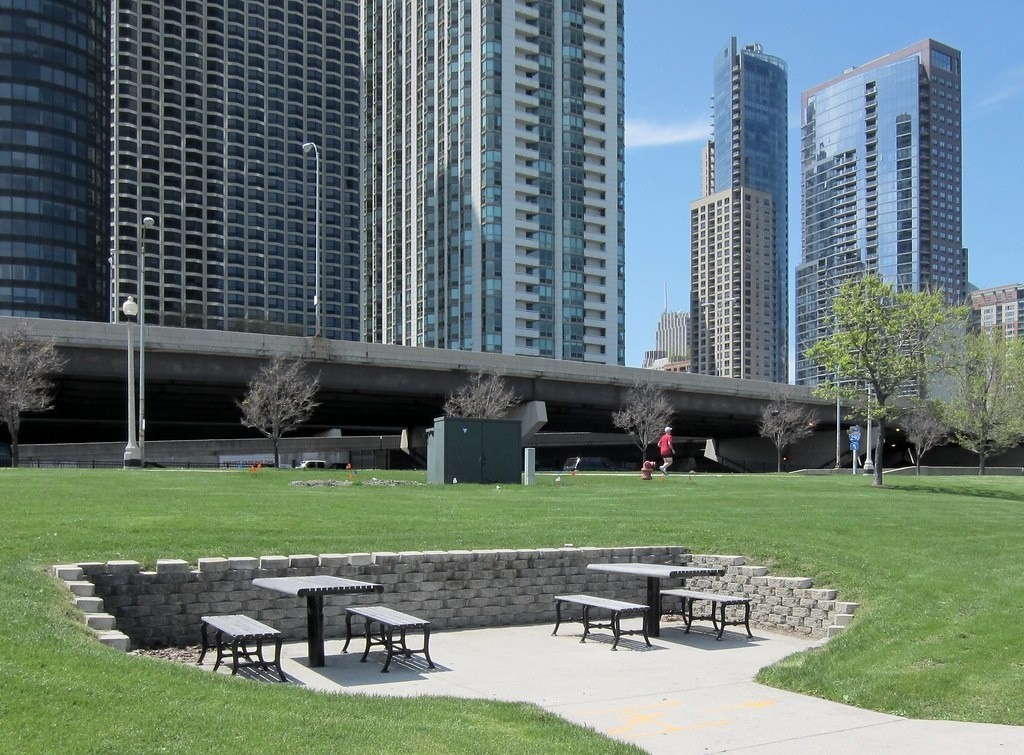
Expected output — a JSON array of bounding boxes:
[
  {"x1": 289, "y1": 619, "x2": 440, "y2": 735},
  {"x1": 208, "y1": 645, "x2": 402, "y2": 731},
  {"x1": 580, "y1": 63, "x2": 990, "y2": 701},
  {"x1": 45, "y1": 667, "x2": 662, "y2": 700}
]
[{"x1": 564, "y1": 456, "x2": 632, "y2": 472}]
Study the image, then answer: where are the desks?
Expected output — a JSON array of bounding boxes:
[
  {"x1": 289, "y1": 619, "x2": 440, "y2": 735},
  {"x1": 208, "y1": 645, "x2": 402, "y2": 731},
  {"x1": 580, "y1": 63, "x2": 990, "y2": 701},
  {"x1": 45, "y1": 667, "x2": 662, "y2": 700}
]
[
  {"x1": 253, "y1": 575, "x2": 384, "y2": 667},
  {"x1": 587, "y1": 563, "x2": 726, "y2": 638}
]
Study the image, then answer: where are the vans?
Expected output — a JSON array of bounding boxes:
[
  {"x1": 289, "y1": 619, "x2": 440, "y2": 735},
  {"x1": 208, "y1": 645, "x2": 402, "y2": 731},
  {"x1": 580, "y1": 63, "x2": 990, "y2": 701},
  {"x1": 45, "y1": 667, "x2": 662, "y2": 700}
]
[
  {"x1": 295, "y1": 459, "x2": 327, "y2": 471},
  {"x1": 326, "y1": 462, "x2": 349, "y2": 470}
]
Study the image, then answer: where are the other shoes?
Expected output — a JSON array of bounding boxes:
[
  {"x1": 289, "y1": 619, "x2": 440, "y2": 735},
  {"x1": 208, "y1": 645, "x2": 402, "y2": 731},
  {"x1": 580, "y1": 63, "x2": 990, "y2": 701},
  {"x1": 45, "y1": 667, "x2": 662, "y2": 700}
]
[
  {"x1": 659, "y1": 466, "x2": 666, "y2": 474},
  {"x1": 662, "y1": 473, "x2": 669, "y2": 476}
]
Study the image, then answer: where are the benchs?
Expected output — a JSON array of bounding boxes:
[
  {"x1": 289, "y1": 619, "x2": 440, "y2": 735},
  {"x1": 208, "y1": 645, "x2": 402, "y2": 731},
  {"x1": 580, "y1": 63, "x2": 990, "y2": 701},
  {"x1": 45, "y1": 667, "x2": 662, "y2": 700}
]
[
  {"x1": 197, "y1": 614, "x2": 287, "y2": 682},
  {"x1": 340, "y1": 606, "x2": 436, "y2": 673},
  {"x1": 551, "y1": 594, "x2": 652, "y2": 651},
  {"x1": 659, "y1": 589, "x2": 753, "y2": 640}
]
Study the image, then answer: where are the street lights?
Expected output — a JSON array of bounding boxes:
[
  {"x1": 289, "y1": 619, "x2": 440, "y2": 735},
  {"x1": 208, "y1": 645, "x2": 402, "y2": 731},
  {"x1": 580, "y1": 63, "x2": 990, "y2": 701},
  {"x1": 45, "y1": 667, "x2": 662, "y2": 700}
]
[
  {"x1": 139, "y1": 215, "x2": 154, "y2": 466},
  {"x1": 301, "y1": 140, "x2": 325, "y2": 337},
  {"x1": 123, "y1": 297, "x2": 144, "y2": 467}
]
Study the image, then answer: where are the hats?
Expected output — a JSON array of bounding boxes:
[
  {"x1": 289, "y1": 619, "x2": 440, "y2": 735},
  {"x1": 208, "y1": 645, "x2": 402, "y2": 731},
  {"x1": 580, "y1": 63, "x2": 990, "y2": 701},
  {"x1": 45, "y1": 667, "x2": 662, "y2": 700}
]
[{"x1": 665, "y1": 427, "x2": 672, "y2": 432}]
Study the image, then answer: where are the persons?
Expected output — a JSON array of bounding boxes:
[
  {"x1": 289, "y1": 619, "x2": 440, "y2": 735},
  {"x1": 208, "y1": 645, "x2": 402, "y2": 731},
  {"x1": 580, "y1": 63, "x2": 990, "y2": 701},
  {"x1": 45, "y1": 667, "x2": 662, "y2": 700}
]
[{"x1": 658, "y1": 427, "x2": 675, "y2": 475}]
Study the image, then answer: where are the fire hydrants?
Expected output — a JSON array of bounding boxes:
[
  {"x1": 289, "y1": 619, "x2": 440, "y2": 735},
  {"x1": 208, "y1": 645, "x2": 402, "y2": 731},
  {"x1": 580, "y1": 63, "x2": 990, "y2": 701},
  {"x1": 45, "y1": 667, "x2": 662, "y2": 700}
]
[{"x1": 641, "y1": 461, "x2": 657, "y2": 479}]
